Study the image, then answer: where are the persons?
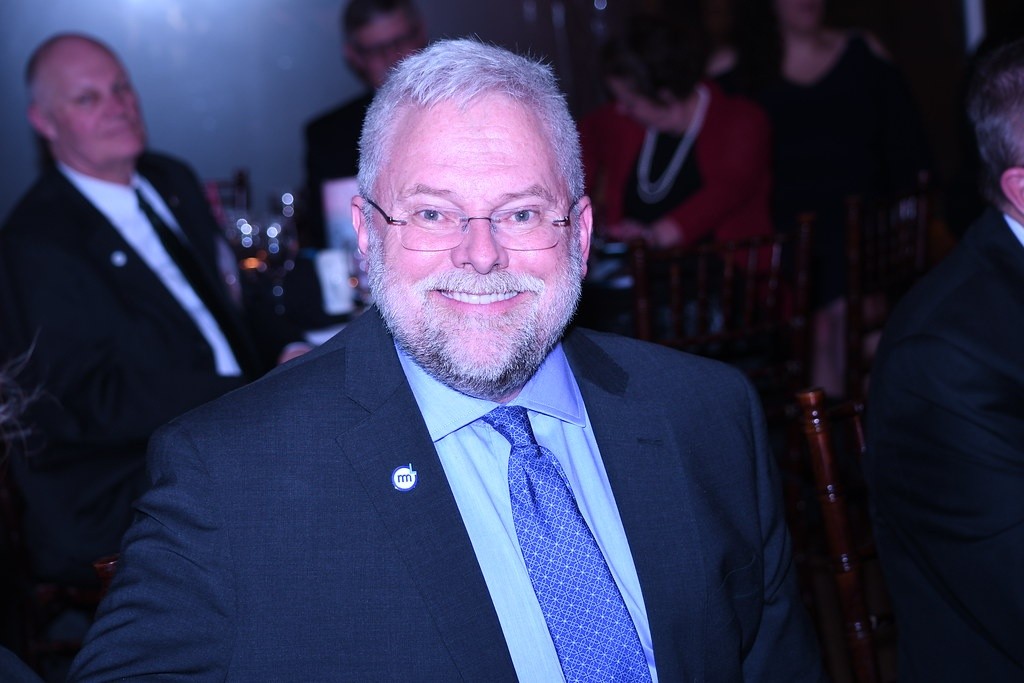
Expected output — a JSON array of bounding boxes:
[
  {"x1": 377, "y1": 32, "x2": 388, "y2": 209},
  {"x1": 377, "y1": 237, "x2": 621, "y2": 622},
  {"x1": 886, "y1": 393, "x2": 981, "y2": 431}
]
[
  {"x1": 0, "y1": 33, "x2": 318, "y2": 683},
  {"x1": 292, "y1": 0, "x2": 431, "y2": 262},
  {"x1": 573, "y1": 20, "x2": 779, "y2": 298},
  {"x1": 842, "y1": 66, "x2": 1024, "y2": 683},
  {"x1": 708, "y1": 0, "x2": 894, "y2": 215},
  {"x1": 69, "y1": 40, "x2": 824, "y2": 683}
]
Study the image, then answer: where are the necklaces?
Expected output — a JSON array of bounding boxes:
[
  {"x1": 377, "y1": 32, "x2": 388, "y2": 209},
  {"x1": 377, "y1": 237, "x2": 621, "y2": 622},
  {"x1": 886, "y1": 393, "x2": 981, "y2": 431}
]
[{"x1": 634, "y1": 83, "x2": 711, "y2": 204}]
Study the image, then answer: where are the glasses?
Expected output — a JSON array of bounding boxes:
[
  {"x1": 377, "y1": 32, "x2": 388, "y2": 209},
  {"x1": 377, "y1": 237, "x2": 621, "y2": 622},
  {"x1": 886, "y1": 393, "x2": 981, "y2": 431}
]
[{"x1": 359, "y1": 192, "x2": 576, "y2": 250}]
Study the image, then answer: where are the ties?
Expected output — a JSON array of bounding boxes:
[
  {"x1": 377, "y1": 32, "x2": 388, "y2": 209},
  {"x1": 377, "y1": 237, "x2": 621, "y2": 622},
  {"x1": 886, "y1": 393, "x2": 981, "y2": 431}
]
[
  {"x1": 136, "y1": 191, "x2": 248, "y2": 373},
  {"x1": 484, "y1": 406, "x2": 653, "y2": 682}
]
